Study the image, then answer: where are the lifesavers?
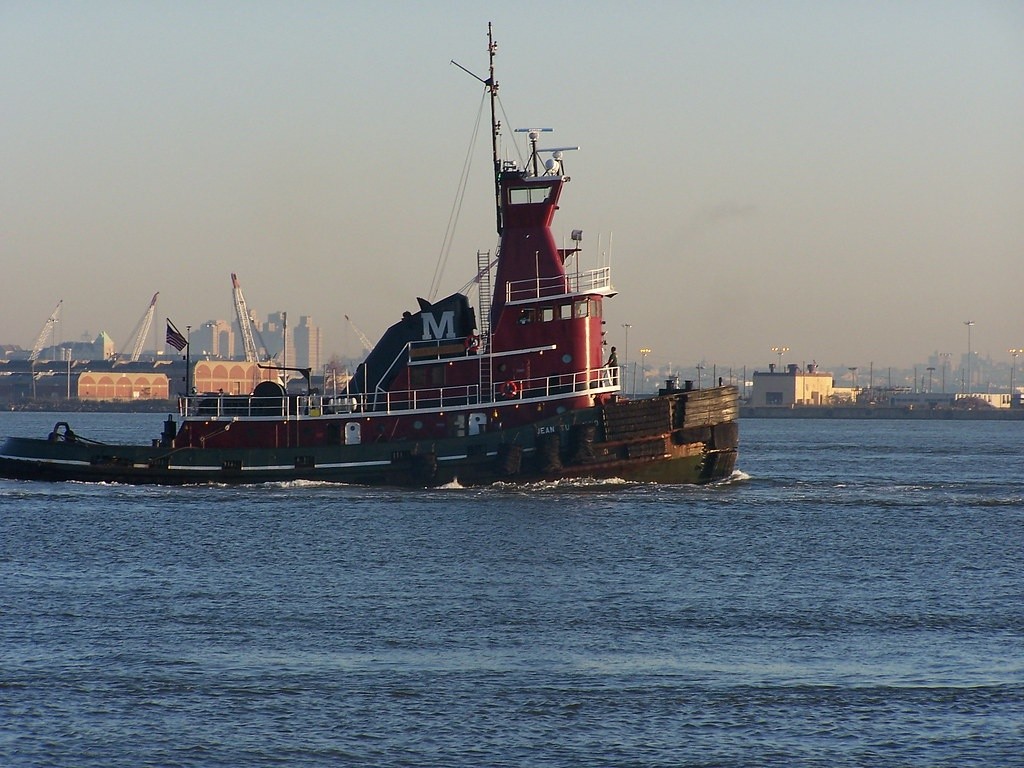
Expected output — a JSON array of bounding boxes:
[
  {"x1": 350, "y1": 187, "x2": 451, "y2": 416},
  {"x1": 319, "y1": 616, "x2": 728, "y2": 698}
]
[
  {"x1": 499, "y1": 380, "x2": 517, "y2": 398},
  {"x1": 465, "y1": 335, "x2": 480, "y2": 352}
]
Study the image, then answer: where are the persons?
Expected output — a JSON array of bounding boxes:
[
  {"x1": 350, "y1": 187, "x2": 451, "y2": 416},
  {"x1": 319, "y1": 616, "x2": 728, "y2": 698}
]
[
  {"x1": 218, "y1": 388, "x2": 226, "y2": 397},
  {"x1": 603, "y1": 346, "x2": 618, "y2": 385}
]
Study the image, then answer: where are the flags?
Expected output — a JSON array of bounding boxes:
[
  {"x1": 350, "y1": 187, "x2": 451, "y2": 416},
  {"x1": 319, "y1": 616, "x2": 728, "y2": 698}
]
[{"x1": 165, "y1": 324, "x2": 187, "y2": 352}]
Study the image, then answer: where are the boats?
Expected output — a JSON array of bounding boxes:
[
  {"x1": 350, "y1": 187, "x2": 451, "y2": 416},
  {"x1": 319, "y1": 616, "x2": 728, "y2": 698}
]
[{"x1": 0, "y1": 18, "x2": 740, "y2": 484}]
